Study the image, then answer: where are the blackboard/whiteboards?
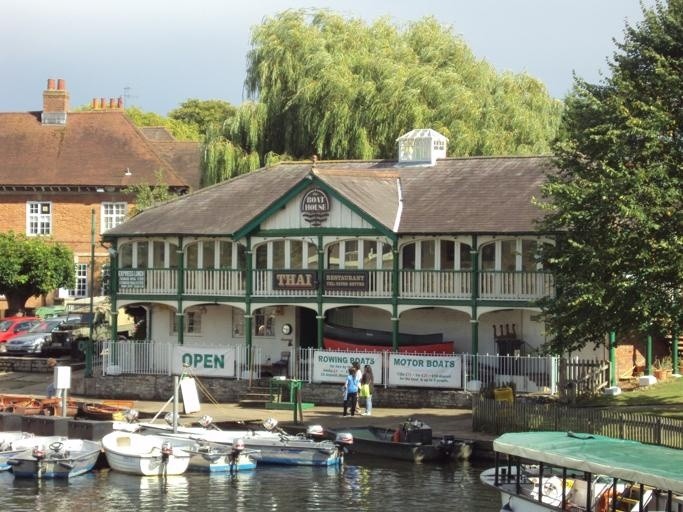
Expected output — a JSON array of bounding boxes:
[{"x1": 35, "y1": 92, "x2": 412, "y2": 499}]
[{"x1": 180, "y1": 377, "x2": 202, "y2": 414}]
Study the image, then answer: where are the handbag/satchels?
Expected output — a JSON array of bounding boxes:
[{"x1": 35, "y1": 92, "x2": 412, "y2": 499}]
[{"x1": 359, "y1": 383, "x2": 370, "y2": 398}]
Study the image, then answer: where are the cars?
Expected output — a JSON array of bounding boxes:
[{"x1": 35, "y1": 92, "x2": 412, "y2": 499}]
[{"x1": 0, "y1": 316, "x2": 81, "y2": 355}]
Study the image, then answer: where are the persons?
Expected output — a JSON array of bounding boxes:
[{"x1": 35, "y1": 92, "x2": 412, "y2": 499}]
[{"x1": 343, "y1": 362, "x2": 373, "y2": 416}]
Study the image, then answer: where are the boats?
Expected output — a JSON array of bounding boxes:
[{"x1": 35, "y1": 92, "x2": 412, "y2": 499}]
[
  {"x1": 80, "y1": 401, "x2": 140, "y2": 424},
  {"x1": 7, "y1": 438, "x2": 102, "y2": 478},
  {"x1": 0, "y1": 430, "x2": 36, "y2": 472},
  {"x1": 139, "y1": 412, "x2": 356, "y2": 473},
  {"x1": 325, "y1": 416, "x2": 480, "y2": 462},
  {"x1": 480, "y1": 432, "x2": 683, "y2": 512},
  {"x1": 323, "y1": 320, "x2": 454, "y2": 351},
  {"x1": 0, "y1": 394, "x2": 85, "y2": 418},
  {"x1": 100, "y1": 430, "x2": 192, "y2": 478}
]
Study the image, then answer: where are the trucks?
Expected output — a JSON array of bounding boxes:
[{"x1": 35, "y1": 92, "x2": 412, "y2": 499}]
[{"x1": 41, "y1": 295, "x2": 136, "y2": 358}]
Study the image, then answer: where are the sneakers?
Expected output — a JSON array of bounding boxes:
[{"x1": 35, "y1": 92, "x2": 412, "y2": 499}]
[{"x1": 343, "y1": 410, "x2": 371, "y2": 416}]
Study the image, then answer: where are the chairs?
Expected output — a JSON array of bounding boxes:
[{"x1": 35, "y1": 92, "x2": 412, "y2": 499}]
[{"x1": 272, "y1": 351, "x2": 289, "y2": 378}]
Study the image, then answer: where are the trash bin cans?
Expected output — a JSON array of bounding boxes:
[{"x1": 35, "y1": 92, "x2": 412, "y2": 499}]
[{"x1": 494, "y1": 386, "x2": 514, "y2": 418}]
